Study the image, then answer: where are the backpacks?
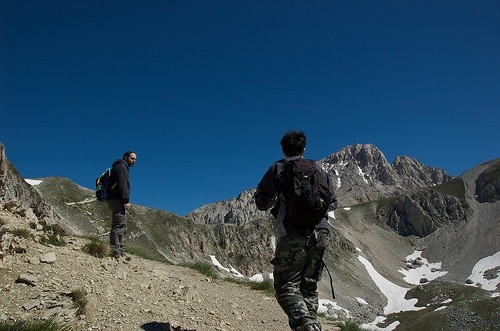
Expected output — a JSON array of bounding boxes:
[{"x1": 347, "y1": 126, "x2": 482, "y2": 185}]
[
  {"x1": 96, "y1": 168, "x2": 111, "y2": 202},
  {"x1": 279, "y1": 158, "x2": 331, "y2": 228}
]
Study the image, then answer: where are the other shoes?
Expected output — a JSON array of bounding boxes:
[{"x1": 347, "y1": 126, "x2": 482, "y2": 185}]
[{"x1": 111, "y1": 253, "x2": 131, "y2": 261}]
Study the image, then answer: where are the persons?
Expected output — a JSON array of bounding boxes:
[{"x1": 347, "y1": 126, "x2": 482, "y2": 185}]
[
  {"x1": 255, "y1": 130, "x2": 338, "y2": 331},
  {"x1": 106, "y1": 151, "x2": 137, "y2": 261}
]
